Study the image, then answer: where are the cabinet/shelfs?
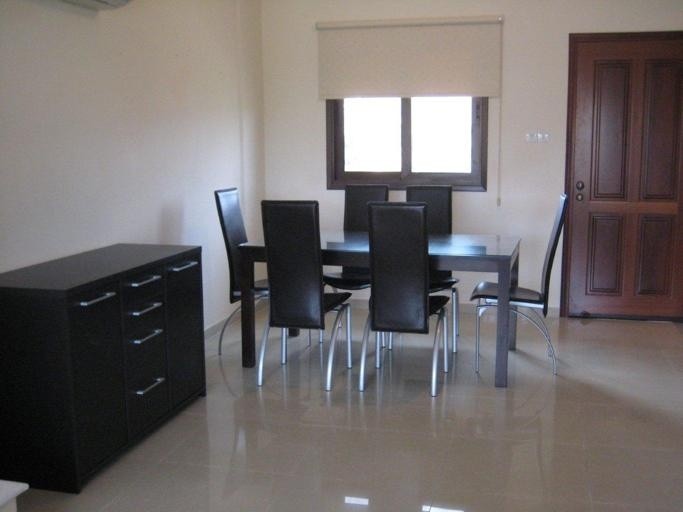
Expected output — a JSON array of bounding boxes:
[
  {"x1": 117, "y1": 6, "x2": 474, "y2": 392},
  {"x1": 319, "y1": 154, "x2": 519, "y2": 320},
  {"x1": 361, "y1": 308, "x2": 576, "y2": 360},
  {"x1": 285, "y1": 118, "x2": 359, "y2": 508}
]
[{"x1": 0, "y1": 244, "x2": 208, "y2": 494}]
